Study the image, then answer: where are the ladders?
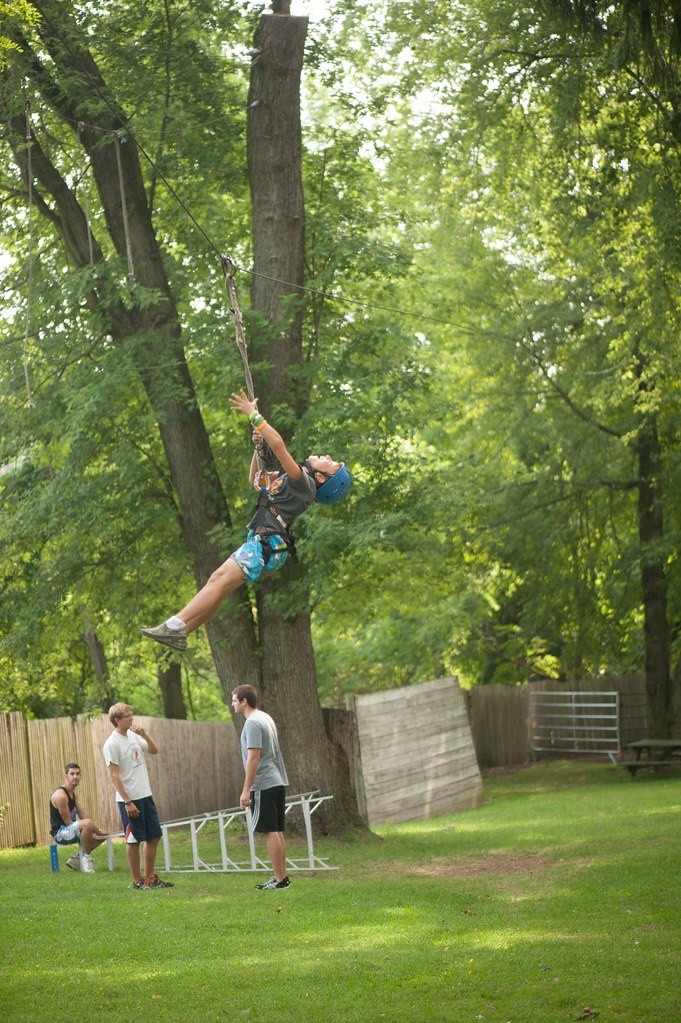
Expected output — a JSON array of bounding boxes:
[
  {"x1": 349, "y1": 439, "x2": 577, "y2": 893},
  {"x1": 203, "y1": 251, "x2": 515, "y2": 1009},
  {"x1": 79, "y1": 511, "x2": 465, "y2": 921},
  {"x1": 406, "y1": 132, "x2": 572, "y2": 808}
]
[{"x1": 95, "y1": 789, "x2": 343, "y2": 873}]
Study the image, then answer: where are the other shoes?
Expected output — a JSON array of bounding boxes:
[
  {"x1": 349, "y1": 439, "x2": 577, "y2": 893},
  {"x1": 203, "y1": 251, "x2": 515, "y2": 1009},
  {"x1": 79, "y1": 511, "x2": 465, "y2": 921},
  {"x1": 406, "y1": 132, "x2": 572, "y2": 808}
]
[
  {"x1": 142, "y1": 622, "x2": 187, "y2": 639},
  {"x1": 153, "y1": 638, "x2": 187, "y2": 651}
]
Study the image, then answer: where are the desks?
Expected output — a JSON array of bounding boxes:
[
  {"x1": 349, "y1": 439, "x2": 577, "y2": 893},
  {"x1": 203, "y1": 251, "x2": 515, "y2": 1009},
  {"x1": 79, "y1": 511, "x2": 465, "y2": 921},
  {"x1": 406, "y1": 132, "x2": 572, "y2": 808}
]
[{"x1": 629, "y1": 739, "x2": 681, "y2": 778}]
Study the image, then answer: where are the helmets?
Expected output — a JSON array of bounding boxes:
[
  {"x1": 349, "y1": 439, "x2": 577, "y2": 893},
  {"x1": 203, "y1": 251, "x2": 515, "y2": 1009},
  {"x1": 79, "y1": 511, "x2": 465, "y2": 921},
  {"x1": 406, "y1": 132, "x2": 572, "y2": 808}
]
[{"x1": 314, "y1": 462, "x2": 351, "y2": 505}]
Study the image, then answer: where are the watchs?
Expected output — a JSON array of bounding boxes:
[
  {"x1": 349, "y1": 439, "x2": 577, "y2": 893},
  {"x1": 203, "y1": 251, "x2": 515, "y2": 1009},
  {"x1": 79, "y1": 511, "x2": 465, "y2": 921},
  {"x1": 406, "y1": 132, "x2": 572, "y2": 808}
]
[{"x1": 125, "y1": 800, "x2": 133, "y2": 805}]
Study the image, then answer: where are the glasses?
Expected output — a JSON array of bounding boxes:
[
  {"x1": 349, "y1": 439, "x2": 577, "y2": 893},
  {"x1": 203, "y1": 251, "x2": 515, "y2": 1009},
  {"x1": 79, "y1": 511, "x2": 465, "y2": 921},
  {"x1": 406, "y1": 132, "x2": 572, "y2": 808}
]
[{"x1": 122, "y1": 713, "x2": 133, "y2": 718}]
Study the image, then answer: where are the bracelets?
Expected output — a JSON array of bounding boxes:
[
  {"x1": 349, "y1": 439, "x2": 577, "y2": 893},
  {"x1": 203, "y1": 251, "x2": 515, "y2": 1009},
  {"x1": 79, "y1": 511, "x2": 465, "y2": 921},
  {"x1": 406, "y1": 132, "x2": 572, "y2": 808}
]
[
  {"x1": 142, "y1": 732, "x2": 148, "y2": 739},
  {"x1": 249, "y1": 410, "x2": 268, "y2": 431}
]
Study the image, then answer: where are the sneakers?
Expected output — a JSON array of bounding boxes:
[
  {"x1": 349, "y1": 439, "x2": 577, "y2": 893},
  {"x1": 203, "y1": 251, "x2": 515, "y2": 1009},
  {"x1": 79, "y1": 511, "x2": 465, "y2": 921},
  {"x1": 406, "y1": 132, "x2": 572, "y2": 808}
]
[
  {"x1": 143, "y1": 874, "x2": 174, "y2": 888},
  {"x1": 79, "y1": 849, "x2": 95, "y2": 873},
  {"x1": 133, "y1": 876, "x2": 163, "y2": 890},
  {"x1": 66, "y1": 853, "x2": 80, "y2": 871},
  {"x1": 255, "y1": 876, "x2": 291, "y2": 890}
]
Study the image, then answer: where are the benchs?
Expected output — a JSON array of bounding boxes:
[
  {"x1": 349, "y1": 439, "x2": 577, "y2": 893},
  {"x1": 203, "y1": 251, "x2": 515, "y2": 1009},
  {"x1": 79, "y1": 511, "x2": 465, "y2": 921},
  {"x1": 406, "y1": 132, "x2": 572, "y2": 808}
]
[{"x1": 616, "y1": 751, "x2": 681, "y2": 777}]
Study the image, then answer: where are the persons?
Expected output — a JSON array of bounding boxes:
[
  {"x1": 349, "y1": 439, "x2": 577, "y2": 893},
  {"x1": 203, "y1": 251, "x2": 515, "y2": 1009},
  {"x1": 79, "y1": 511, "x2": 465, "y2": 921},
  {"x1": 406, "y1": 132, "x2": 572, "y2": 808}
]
[
  {"x1": 231, "y1": 684, "x2": 291, "y2": 890},
  {"x1": 142, "y1": 389, "x2": 351, "y2": 651},
  {"x1": 103, "y1": 702, "x2": 174, "y2": 890},
  {"x1": 50, "y1": 764, "x2": 109, "y2": 873}
]
